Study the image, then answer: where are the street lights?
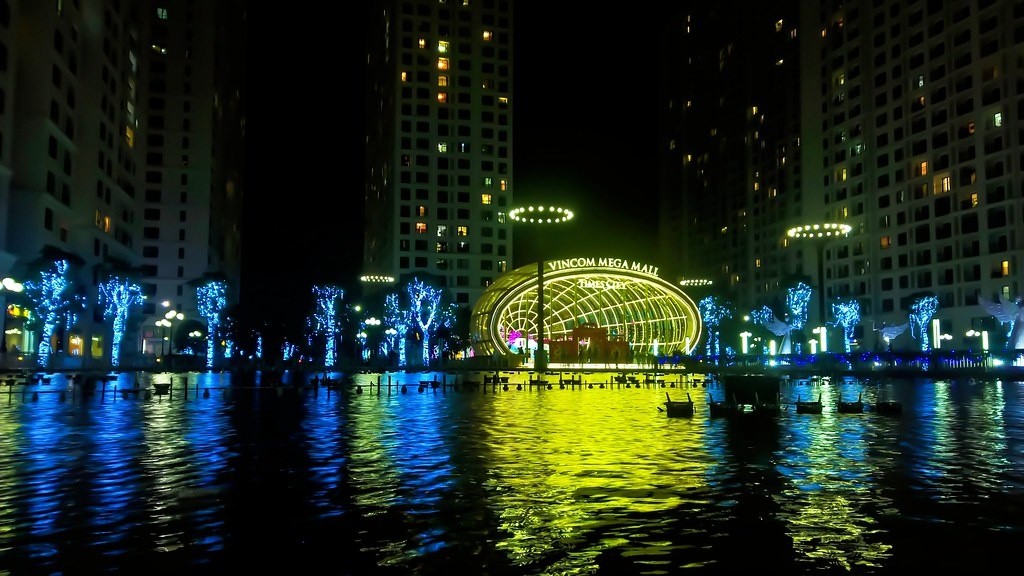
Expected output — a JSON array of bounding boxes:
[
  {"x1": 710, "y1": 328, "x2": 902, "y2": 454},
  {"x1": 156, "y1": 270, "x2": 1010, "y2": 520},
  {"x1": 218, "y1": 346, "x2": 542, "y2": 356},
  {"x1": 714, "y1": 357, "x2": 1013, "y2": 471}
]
[
  {"x1": 786, "y1": 222, "x2": 853, "y2": 352},
  {"x1": 0, "y1": 277, "x2": 400, "y2": 371},
  {"x1": 509, "y1": 205, "x2": 575, "y2": 370}
]
